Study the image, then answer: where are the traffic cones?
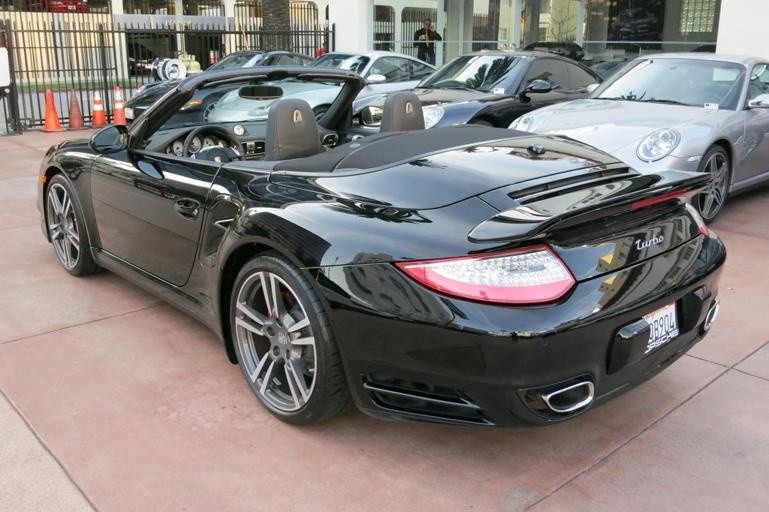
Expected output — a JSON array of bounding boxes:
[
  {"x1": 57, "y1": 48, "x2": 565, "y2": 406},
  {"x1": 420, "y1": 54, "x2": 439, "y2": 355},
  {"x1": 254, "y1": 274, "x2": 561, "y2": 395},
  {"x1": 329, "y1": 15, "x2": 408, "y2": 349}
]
[
  {"x1": 87, "y1": 86, "x2": 109, "y2": 130},
  {"x1": 109, "y1": 83, "x2": 127, "y2": 127},
  {"x1": 65, "y1": 88, "x2": 90, "y2": 131},
  {"x1": 38, "y1": 87, "x2": 66, "y2": 134}
]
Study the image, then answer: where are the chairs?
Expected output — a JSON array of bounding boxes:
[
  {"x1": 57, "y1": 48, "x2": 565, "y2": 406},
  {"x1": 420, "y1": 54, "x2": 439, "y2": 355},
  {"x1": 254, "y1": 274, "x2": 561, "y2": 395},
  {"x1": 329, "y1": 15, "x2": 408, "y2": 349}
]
[
  {"x1": 378, "y1": 90, "x2": 428, "y2": 134},
  {"x1": 259, "y1": 97, "x2": 325, "y2": 161}
]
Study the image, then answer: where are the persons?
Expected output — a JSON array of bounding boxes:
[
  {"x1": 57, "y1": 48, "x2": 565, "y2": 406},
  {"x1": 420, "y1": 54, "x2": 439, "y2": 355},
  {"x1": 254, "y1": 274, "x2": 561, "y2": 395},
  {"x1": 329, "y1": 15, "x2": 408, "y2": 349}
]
[
  {"x1": 411, "y1": 19, "x2": 443, "y2": 65},
  {"x1": 316, "y1": 41, "x2": 326, "y2": 57}
]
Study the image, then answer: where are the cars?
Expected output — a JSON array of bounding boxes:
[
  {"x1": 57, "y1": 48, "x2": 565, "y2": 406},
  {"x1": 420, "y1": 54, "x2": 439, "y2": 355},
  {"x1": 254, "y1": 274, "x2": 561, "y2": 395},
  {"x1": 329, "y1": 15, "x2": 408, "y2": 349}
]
[
  {"x1": 507, "y1": 51, "x2": 768, "y2": 225},
  {"x1": 367, "y1": 47, "x2": 617, "y2": 141},
  {"x1": 21, "y1": 0, "x2": 91, "y2": 13},
  {"x1": 207, "y1": 49, "x2": 443, "y2": 145},
  {"x1": 123, "y1": 49, "x2": 319, "y2": 122}
]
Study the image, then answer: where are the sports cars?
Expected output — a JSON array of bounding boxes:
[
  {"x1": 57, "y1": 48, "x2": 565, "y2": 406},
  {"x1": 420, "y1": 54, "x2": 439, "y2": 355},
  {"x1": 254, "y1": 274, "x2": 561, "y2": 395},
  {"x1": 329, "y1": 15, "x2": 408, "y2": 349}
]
[{"x1": 36, "y1": 63, "x2": 731, "y2": 432}]
